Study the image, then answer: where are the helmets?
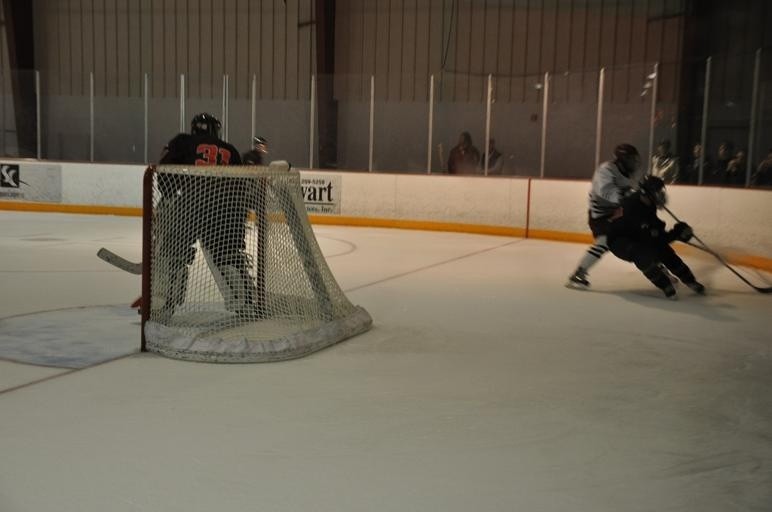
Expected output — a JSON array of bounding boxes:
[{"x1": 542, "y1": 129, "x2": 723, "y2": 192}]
[
  {"x1": 190, "y1": 111, "x2": 222, "y2": 137},
  {"x1": 254, "y1": 136, "x2": 269, "y2": 157},
  {"x1": 614, "y1": 143, "x2": 641, "y2": 171},
  {"x1": 639, "y1": 175, "x2": 666, "y2": 209}
]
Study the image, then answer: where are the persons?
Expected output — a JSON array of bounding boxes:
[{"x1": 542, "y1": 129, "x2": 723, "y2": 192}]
[
  {"x1": 652, "y1": 138, "x2": 772, "y2": 192},
  {"x1": 607, "y1": 174, "x2": 707, "y2": 297},
  {"x1": 242, "y1": 134, "x2": 267, "y2": 166},
  {"x1": 142, "y1": 112, "x2": 259, "y2": 319},
  {"x1": 478, "y1": 138, "x2": 505, "y2": 176},
  {"x1": 449, "y1": 131, "x2": 481, "y2": 176},
  {"x1": 567, "y1": 142, "x2": 641, "y2": 287}
]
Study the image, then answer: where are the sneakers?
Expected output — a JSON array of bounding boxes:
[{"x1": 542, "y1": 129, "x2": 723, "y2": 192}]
[
  {"x1": 657, "y1": 267, "x2": 704, "y2": 297},
  {"x1": 570, "y1": 267, "x2": 590, "y2": 286}
]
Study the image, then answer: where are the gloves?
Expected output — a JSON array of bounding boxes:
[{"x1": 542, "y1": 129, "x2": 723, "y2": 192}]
[{"x1": 675, "y1": 220, "x2": 693, "y2": 242}]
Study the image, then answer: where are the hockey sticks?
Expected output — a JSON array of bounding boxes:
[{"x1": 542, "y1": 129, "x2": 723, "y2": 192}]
[
  {"x1": 97, "y1": 248, "x2": 143, "y2": 274},
  {"x1": 664, "y1": 207, "x2": 772, "y2": 294}
]
